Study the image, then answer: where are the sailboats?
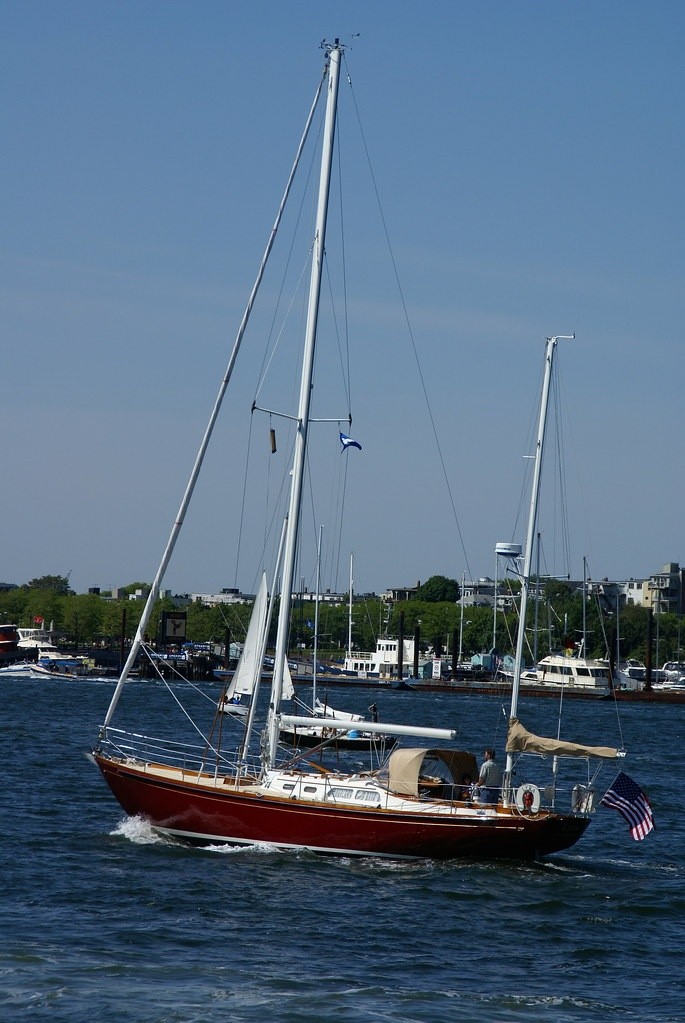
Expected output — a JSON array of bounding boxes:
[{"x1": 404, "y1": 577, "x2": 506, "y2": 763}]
[
  {"x1": 278, "y1": 525, "x2": 398, "y2": 752},
  {"x1": 312, "y1": 533, "x2": 685, "y2": 690},
  {"x1": 86, "y1": 38, "x2": 628, "y2": 865},
  {"x1": 216, "y1": 568, "x2": 268, "y2": 716}
]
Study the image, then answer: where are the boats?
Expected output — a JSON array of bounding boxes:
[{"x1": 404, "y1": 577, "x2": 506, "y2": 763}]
[
  {"x1": 30, "y1": 660, "x2": 83, "y2": 682},
  {"x1": 0, "y1": 660, "x2": 42, "y2": 677},
  {"x1": 0, "y1": 620, "x2": 77, "y2": 674}
]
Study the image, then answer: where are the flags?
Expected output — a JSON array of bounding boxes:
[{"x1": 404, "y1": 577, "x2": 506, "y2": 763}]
[
  {"x1": 598, "y1": 771, "x2": 657, "y2": 842},
  {"x1": 306, "y1": 619, "x2": 315, "y2": 629},
  {"x1": 34, "y1": 615, "x2": 42, "y2": 623}
]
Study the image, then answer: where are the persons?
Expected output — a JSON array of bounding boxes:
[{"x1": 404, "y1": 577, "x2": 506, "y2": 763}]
[
  {"x1": 233, "y1": 696, "x2": 239, "y2": 704},
  {"x1": 478, "y1": 747, "x2": 504, "y2": 804}
]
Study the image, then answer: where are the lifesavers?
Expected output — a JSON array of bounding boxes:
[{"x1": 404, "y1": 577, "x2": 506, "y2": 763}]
[{"x1": 516, "y1": 783, "x2": 541, "y2": 813}]
